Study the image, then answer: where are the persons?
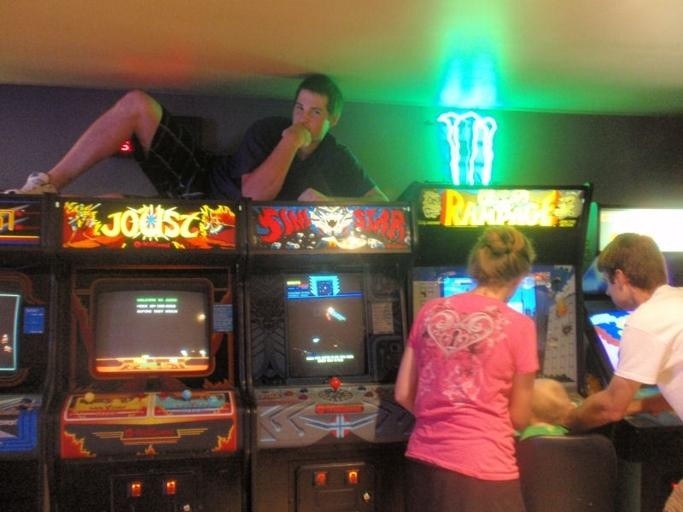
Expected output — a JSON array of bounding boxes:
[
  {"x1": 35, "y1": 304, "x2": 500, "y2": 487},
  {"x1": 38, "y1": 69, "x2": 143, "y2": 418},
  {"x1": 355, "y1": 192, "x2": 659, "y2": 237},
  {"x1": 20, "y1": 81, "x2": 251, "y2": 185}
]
[
  {"x1": 1, "y1": 71, "x2": 390, "y2": 206},
  {"x1": 392, "y1": 225, "x2": 540, "y2": 512},
  {"x1": 567, "y1": 232, "x2": 683, "y2": 511},
  {"x1": 514, "y1": 378, "x2": 576, "y2": 441}
]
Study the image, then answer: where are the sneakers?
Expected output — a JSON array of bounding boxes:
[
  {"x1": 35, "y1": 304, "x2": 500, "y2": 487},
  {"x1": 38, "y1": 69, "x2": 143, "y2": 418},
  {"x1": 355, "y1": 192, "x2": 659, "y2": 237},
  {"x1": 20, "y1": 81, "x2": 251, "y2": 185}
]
[{"x1": 0, "y1": 171, "x2": 58, "y2": 197}]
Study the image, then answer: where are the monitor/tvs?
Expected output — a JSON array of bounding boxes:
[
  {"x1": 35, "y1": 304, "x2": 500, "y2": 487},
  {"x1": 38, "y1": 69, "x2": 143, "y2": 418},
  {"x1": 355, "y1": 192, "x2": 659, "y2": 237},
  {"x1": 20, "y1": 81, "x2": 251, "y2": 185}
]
[
  {"x1": 274, "y1": 267, "x2": 370, "y2": 384},
  {"x1": 85, "y1": 275, "x2": 214, "y2": 383},
  {"x1": 589, "y1": 305, "x2": 660, "y2": 399},
  {"x1": 0, "y1": 291, "x2": 28, "y2": 382},
  {"x1": 439, "y1": 271, "x2": 537, "y2": 332}
]
